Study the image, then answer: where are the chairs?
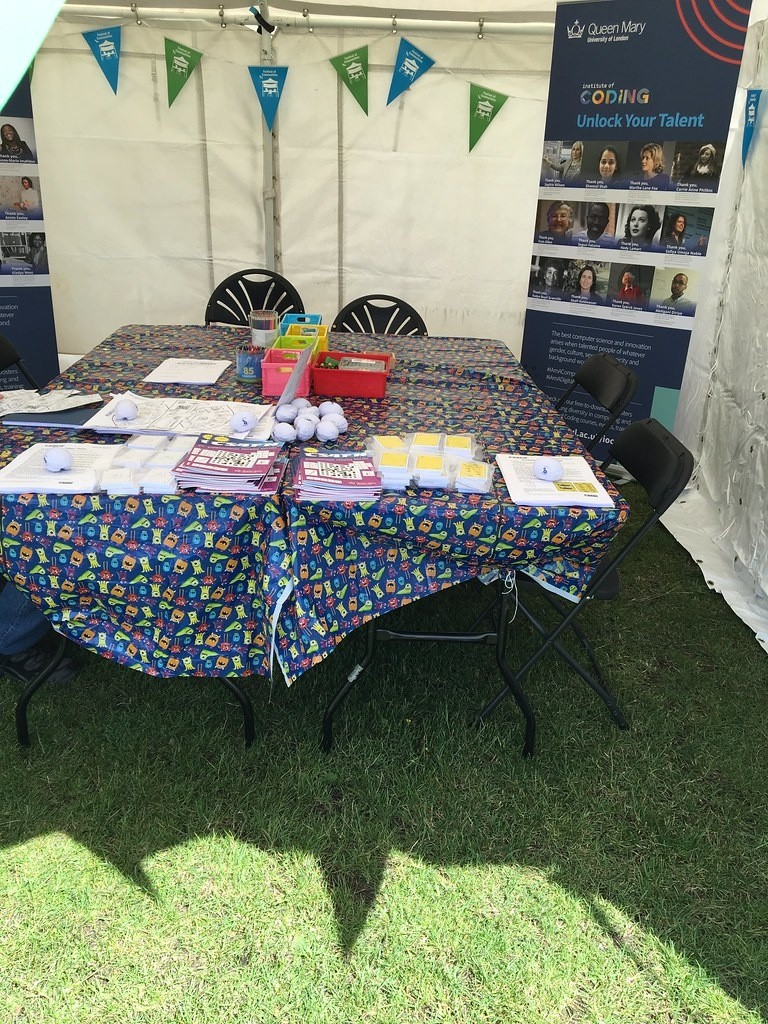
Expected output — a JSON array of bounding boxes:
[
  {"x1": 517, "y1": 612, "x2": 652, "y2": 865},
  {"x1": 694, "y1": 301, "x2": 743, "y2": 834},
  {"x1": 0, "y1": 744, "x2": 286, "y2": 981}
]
[
  {"x1": 204, "y1": 267, "x2": 307, "y2": 327},
  {"x1": 330, "y1": 292, "x2": 430, "y2": 337},
  {"x1": 466, "y1": 416, "x2": 694, "y2": 735},
  {"x1": 0, "y1": 331, "x2": 41, "y2": 391},
  {"x1": 554, "y1": 351, "x2": 636, "y2": 450}
]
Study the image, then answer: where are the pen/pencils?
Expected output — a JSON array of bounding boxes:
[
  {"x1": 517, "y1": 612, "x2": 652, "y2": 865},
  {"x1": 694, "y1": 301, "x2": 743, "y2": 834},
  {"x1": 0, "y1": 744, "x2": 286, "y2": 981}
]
[
  {"x1": 236, "y1": 345, "x2": 264, "y2": 370},
  {"x1": 248, "y1": 310, "x2": 278, "y2": 330}
]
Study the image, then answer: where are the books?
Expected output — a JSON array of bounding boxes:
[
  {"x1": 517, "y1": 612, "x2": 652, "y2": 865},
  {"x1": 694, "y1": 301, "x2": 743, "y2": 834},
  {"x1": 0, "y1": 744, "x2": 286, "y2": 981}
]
[
  {"x1": 290, "y1": 445, "x2": 382, "y2": 501},
  {"x1": 1, "y1": 408, "x2": 100, "y2": 429},
  {"x1": 171, "y1": 432, "x2": 290, "y2": 494}
]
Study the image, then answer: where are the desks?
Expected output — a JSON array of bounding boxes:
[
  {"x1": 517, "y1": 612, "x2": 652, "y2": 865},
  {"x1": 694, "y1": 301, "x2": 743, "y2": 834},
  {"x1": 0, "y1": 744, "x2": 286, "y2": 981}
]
[{"x1": 0, "y1": 323, "x2": 629, "y2": 761}]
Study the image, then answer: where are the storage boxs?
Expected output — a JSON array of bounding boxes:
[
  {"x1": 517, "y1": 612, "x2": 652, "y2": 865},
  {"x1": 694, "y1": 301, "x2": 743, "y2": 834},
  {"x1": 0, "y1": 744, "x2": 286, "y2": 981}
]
[
  {"x1": 285, "y1": 324, "x2": 329, "y2": 354},
  {"x1": 278, "y1": 313, "x2": 322, "y2": 336},
  {"x1": 308, "y1": 350, "x2": 390, "y2": 397},
  {"x1": 272, "y1": 336, "x2": 320, "y2": 360},
  {"x1": 261, "y1": 350, "x2": 314, "y2": 396}
]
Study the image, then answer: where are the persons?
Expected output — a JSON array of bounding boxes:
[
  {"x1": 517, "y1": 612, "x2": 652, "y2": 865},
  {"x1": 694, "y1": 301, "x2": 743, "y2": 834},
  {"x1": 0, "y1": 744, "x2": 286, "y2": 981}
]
[
  {"x1": 663, "y1": 273, "x2": 693, "y2": 310},
  {"x1": 617, "y1": 205, "x2": 661, "y2": 251},
  {"x1": 0, "y1": 124, "x2": 33, "y2": 161},
  {"x1": 24, "y1": 233, "x2": 48, "y2": 274},
  {"x1": 663, "y1": 213, "x2": 707, "y2": 252},
  {"x1": 597, "y1": 146, "x2": 622, "y2": 185},
  {"x1": 533, "y1": 259, "x2": 565, "y2": 296},
  {"x1": 639, "y1": 143, "x2": 669, "y2": 185},
  {"x1": 543, "y1": 141, "x2": 583, "y2": 184},
  {"x1": 14, "y1": 176, "x2": 39, "y2": 211},
  {"x1": 689, "y1": 144, "x2": 720, "y2": 188},
  {"x1": 578, "y1": 202, "x2": 613, "y2": 247},
  {"x1": 537, "y1": 200, "x2": 576, "y2": 245},
  {"x1": 569, "y1": 265, "x2": 603, "y2": 304},
  {"x1": 617, "y1": 272, "x2": 644, "y2": 306}
]
